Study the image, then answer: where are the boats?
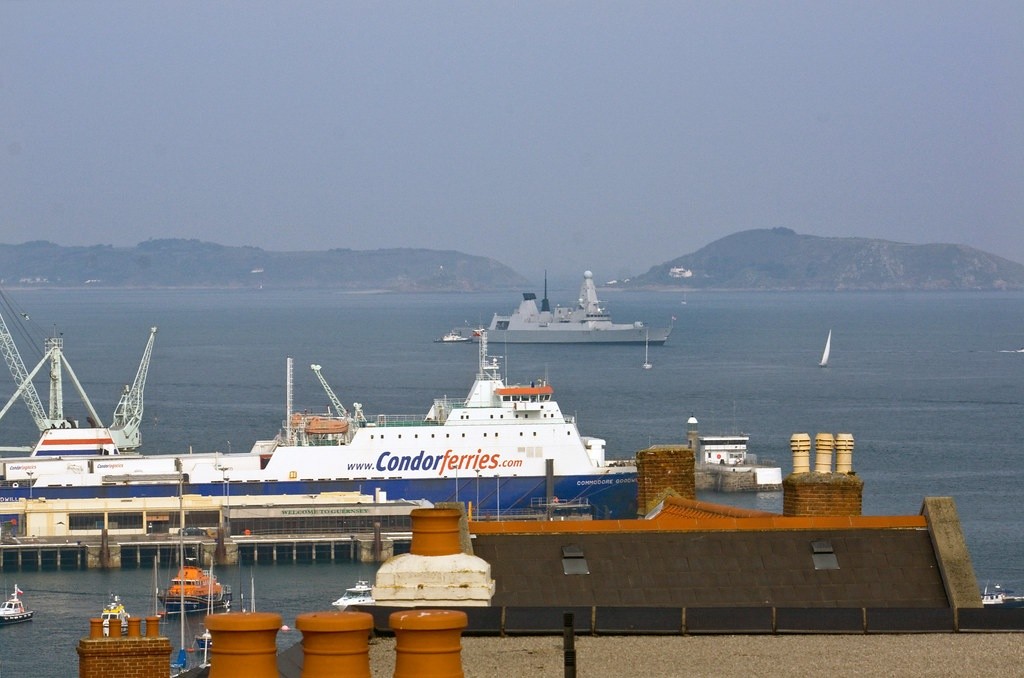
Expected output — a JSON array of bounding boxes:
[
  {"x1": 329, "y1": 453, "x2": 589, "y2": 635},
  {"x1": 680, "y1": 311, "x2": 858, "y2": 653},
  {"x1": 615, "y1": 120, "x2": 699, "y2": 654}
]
[
  {"x1": 0, "y1": 584, "x2": 34, "y2": 625},
  {"x1": 431, "y1": 267, "x2": 678, "y2": 346},
  {"x1": 156, "y1": 565, "x2": 232, "y2": 617},
  {"x1": 331, "y1": 582, "x2": 374, "y2": 611},
  {"x1": 193, "y1": 555, "x2": 212, "y2": 651},
  {"x1": 100, "y1": 592, "x2": 131, "y2": 637},
  {"x1": 167, "y1": 470, "x2": 209, "y2": 678},
  {"x1": 0, "y1": 282, "x2": 637, "y2": 555},
  {"x1": 306, "y1": 416, "x2": 348, "y2": 434},
  {"x1": 292, "y1": 411, "x2": 302, "y2": 426}
]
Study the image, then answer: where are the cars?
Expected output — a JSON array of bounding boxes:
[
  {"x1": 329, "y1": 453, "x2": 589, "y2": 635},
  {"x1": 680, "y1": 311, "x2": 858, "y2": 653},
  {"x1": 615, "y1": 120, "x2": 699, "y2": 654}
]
[{"x1": 177, "y1": 526, "x2": 208, "y2": 537}]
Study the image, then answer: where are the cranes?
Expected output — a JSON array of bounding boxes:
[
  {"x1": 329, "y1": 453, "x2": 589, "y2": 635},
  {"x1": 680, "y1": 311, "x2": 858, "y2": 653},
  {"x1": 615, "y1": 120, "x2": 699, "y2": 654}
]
[
  {"x1": 0, "y1": 284, "x2": 157, "y2": 453},
  {"x1": 274, "y1": 355, "x2": 367, "y2": 447}
]
